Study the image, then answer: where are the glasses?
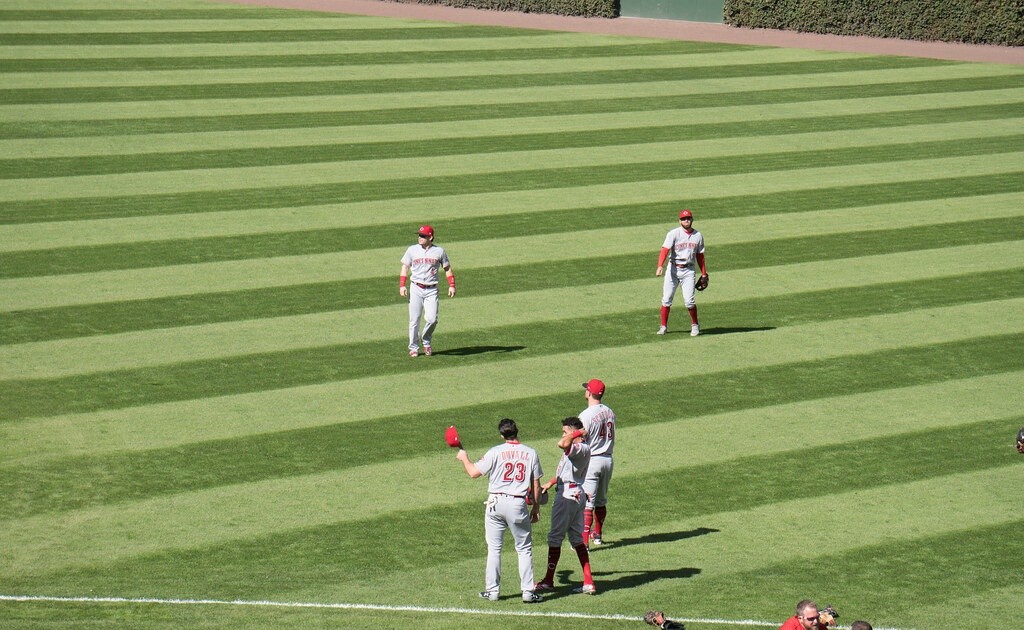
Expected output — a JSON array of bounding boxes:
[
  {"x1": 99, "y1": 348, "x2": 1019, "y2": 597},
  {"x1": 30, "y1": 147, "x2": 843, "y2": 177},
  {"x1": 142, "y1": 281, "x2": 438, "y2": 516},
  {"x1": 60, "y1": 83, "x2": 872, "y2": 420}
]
[
  {"x1": 419, "y1": 234, "x2": 429, "y2": 238},
  {"x1": 801, "y1": 614, "x2": 820, "y2": 621}
]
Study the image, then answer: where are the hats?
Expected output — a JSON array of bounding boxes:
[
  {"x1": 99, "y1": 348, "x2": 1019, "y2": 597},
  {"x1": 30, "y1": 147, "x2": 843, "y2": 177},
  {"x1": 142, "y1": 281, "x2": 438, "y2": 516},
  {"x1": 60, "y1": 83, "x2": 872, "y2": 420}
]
[
  {"x1": 524, "y1": 488, "x2": 549, "y2": 505},
  {"x1": 679, "y1": 209, "x2": 692, "y2": 219},
  {"x1": 446, "y1": 426, "x2": 464, "y2": 450},
  {"x1": 582, "y1": 379, "x2": 605, "y2": 396},
  {"x1": 414, "y1": 225, "x2": 434, "y2": 236}
]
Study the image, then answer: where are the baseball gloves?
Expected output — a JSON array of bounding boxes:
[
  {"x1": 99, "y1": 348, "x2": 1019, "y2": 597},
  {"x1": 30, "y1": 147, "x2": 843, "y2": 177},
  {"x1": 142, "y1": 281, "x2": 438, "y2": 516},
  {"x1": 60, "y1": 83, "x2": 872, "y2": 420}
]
[
  {"x1": 695, "y1": 273, "x2": 709, "y2": 291},
  {"x1": 818, "y1": 604, "x2": 838, "y2": 628},
  {"x1": 644, "y1": 610, "x2": 665, "y2": 627}
]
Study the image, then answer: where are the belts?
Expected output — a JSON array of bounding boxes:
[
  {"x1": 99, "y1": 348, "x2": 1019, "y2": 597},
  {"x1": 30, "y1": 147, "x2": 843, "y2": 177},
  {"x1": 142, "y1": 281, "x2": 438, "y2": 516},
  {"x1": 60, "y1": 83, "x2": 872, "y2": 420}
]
[
  {"x1": 670, "y1": 261, "x2": 693, "y2": 268},
  {"x1": 493, "y1": 493, "x2": 524, "y2": 498},
  {"x1": 412, "y1": 281, "x2": 436, "y2": 289},
  {"x1": 555, "y1": 483, "x2": 579, "y2": 491}
]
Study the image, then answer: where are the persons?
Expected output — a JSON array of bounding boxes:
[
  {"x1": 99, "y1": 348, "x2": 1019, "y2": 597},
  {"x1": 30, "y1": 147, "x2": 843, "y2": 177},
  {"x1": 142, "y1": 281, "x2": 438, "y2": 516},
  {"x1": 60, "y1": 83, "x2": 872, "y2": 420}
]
[
  {"x1": 850, "y1": 620, "x2": 872, "y2": 630},
  {"x1": 534, "y1": 417, "x2": 596, "y2": 595},
  {"x1": 570, "y1": 379, "x2": 615, "y2": 554},
  {"x1": 399, "y1": 225, "x2": 457, "y2": 358},
  {"x1": 656, "y1": 210, "x2": 709, "y2": 336},
  {"x1": 456, "y1": 419, "x2": 544, "y2": 603},
  {"x1": 779, "y1": 600, "x2": 829, "y2": 630}
]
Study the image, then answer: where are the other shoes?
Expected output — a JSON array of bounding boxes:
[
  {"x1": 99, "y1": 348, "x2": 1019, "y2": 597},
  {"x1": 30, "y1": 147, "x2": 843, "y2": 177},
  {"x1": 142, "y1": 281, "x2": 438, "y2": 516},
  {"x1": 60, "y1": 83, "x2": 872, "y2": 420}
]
[
  {"x1": 571, "y1": 543, "x2": 589, "y2": 551},
  {"x1": 589, "y1": 530, "x2": 601, "y2": 545}
]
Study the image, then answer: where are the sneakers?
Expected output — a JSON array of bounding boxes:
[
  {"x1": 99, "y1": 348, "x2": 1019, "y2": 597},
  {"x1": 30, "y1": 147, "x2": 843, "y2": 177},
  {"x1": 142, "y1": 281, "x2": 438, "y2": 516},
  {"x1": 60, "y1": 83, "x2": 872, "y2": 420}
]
[
  {"x1": 573, "y1": 584, "x2": 596, "y2": 595},
  {"x1": 690, "y1": 324, "x2": 700, "y2": 336},
  {"x1": 479, "y1": 591, "x2": 499, "y2": 601},
  {"x1": 422, "y1": 345, "x2": 432, "y2": 355},
  {"x1": 534, "y1": 581, "x2": 554, "y2": 591},
  {"x1": 657, "y1": 325, "x2": 667, "y2": 335},
  {"x1": 410, "y1": 350, "x2": 418, "y2": 357},
  {"x1": 523, "y1": 592, "x2": 543, "y2": 602}
]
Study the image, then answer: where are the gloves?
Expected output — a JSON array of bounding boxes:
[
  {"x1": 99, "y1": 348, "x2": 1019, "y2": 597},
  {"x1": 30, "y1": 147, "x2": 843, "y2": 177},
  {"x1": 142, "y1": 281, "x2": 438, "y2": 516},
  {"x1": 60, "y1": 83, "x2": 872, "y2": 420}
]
[{"x1": 483, "y1": 496, "x2": 498, "y2": 508}]
